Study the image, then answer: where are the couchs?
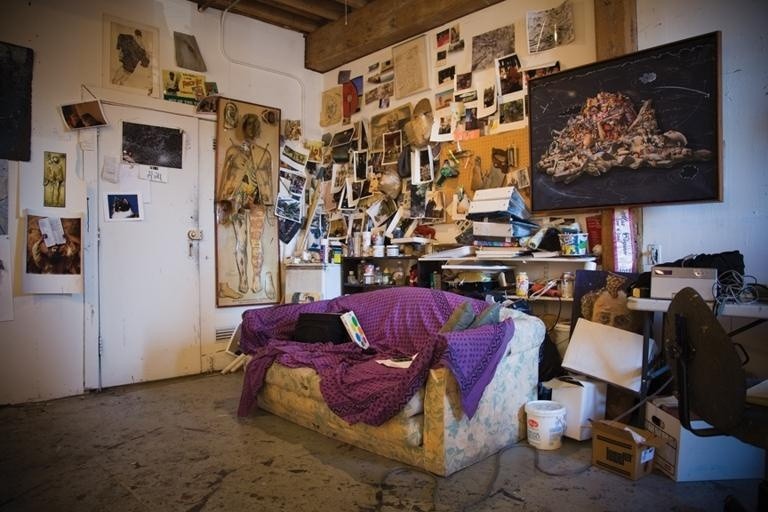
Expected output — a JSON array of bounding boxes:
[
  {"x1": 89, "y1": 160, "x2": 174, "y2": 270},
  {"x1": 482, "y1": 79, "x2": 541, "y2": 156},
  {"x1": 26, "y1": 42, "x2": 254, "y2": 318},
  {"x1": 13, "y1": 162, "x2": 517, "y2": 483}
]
[{"x1": 236, "y1": 286, "x2": 547, "y2": 478}]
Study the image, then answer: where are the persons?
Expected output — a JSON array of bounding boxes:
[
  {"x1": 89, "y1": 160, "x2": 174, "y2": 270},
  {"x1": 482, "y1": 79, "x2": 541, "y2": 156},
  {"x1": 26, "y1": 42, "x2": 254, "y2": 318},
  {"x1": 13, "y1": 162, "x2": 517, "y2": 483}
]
[
  {"x1": 31, "y1": 232, "x2": 80, "y2": 273},
  {"x1": 219, "y1": 113, "x2": 276, "y2": 293},
  {"x1": 166, "y1": 70, "x2": 181, "y2": 95},
  {"x1": 44, "y1": 153, "x2": 64, "y2": 207},
  {"x1": 581, "y1": 276, "x2": 636, "y2": 332}
]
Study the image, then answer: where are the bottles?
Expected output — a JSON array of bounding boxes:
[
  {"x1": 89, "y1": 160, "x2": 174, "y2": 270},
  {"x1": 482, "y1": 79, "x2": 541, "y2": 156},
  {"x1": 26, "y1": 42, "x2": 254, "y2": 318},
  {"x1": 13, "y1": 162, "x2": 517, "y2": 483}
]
[
  {"x1": 348, "y1": 271, "x2": 355, "y2": 283},
  {"x1": 372, "y1": 245, "x2": 400, "y2": 257},
  {"x1": 393, "y1": 227, "x2": 404, "y2": 238},
  {"x1": 396, "y1": 260, "x2": 405, "y2": 285},
  {"x1": 374, "y1": 267, "x2": 382, "y2": 285},
  {"x1": 358, "y1": 260, "x2": 366, "y2": 282},
  {"x1": 383, "y1": 268, "x2": 391, "y2": 284}
]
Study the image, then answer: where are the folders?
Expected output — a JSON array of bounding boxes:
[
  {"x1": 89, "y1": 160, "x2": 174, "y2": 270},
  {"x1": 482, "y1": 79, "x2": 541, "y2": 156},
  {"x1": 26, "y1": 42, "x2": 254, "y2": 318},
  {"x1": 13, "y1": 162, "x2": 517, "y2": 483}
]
[
  {"x1": 473, "y1": 186, "x2": 525, "y2": 209},
  {"x1": 468, "y1": 199, "x2": 529, "y2": 220},
  {"x1": 473, "y1": 222, "x2": 540, "y2": 237}
]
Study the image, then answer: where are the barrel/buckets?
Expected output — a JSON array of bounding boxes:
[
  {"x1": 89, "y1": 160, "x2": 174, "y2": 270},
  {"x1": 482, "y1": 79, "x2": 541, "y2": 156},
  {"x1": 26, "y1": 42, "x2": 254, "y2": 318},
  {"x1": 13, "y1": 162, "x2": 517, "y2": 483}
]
[{"x1": 525, "y1": 400, "x2": 567, "y2": 451}]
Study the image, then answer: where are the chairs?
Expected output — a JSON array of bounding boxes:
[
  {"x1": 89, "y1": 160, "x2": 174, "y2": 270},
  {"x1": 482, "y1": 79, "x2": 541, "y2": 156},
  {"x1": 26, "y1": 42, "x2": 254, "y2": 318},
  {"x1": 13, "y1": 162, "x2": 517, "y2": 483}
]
[{"x1": 662, "y1": 286, "x2": 768, "y2": 512}]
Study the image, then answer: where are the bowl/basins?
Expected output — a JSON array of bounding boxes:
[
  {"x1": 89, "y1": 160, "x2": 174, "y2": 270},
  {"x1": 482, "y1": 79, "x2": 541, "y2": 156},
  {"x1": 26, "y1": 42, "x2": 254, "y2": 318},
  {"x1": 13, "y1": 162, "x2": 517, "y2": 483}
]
[{"x1": 558, "y1": 233, "x2": 589, "y2": 257}]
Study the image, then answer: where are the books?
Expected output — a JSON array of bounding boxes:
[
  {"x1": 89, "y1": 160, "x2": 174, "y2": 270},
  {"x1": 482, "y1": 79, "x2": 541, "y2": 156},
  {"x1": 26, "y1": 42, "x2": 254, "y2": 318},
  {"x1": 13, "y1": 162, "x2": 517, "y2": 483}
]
[{"x1": 469, "y1": 185, "x2": 538, "y2": 258}]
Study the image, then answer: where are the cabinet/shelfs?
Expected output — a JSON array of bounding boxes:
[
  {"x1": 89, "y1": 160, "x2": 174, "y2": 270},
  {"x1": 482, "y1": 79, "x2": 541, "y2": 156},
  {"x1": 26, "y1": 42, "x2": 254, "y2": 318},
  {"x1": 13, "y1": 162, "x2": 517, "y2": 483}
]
[
  {"x1": 341, "y1": 256, "x2": 424, "y2": 293},
  {"x1": 418, "y1": 252, "x2": 601, "y2": 322}
]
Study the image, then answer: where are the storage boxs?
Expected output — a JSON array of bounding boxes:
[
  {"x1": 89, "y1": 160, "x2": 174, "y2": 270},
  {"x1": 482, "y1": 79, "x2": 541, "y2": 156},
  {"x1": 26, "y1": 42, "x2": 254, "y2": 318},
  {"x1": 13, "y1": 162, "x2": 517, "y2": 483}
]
[
  {"x1": 646, "y1": 401, "x2": 767, "y2": 483},
  {"x1": 561, "y1": 318, "x2": 660, "y2": 398},
  {"x1": 543, "y1": 375, "x2": 608, "y2": 440},
  {"x1": 589, "y1": 417, "x2": 654, "y2": 482}
]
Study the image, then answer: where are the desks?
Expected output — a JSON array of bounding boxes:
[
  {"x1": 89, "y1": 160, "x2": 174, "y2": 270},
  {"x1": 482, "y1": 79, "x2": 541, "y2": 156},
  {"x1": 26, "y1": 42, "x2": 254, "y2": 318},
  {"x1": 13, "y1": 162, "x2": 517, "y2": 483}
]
[{"x1": 626, "y1": 297, "x2": 768, "y2": 431}]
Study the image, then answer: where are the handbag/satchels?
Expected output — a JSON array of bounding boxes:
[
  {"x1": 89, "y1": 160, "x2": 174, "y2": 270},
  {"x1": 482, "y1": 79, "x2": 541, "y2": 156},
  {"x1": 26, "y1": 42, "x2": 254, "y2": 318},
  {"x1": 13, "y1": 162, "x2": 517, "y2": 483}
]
[
  {"x1": 294, "y1": 312, "x2": 352, "y2": 346},
  {"x1": 340, "y1": 310, "x2": 370, "y2": 350}
]
